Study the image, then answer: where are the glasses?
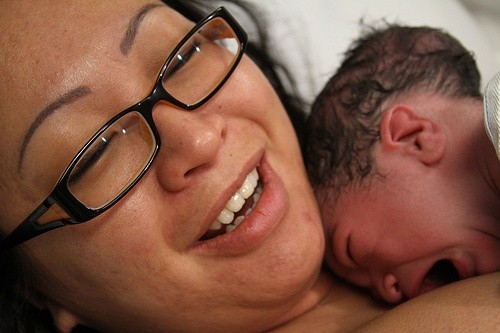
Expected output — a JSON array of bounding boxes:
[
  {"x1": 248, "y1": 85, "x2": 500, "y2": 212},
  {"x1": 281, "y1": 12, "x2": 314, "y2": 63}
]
[{"x1": 3, "y1": 7, "x2": 248, "y2": 252}]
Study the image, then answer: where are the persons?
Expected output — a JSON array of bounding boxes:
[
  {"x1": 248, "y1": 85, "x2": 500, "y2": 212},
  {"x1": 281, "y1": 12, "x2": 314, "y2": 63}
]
[
  {"x1": 0, "y1": 0, "x2": 500, "y2": 332},
  {"x1": 303, "y1": 25, "x2": 500, "y2": 303}
]
